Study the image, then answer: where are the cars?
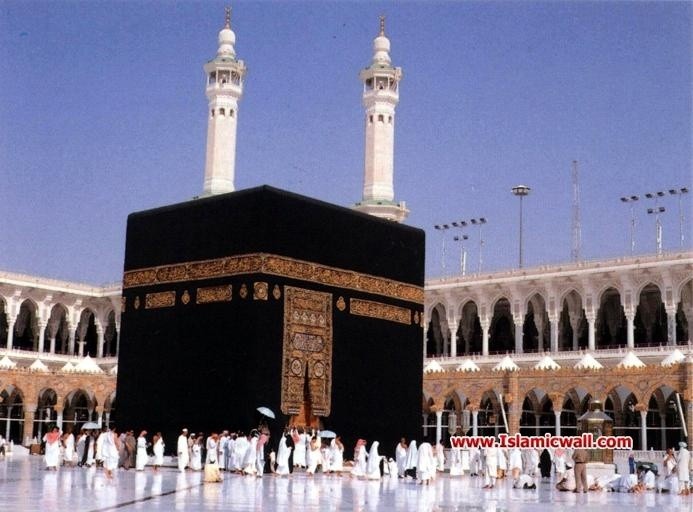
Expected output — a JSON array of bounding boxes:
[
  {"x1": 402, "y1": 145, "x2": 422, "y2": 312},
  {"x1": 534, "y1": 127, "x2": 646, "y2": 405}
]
[{"x1": 631, "y1": 460, "x2": 658, "y2": 477}]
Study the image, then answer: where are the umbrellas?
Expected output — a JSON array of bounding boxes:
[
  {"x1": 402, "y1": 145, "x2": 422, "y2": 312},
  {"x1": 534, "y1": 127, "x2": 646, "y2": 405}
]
[{"x1": 256, "y1": 405, "x2": 275, "y2": 421}]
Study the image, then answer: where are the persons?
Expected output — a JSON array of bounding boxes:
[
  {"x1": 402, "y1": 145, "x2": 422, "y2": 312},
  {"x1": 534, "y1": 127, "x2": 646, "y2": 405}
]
[{"x1": 0, "y1": 421, "x2": 692, "y2": 497}]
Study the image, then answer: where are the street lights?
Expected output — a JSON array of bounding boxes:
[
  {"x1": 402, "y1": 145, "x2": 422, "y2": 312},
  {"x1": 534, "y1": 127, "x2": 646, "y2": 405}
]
[
  {"x1": 514, "y1": 184, "x2": 529, "y2": 270},
  {"x1": 434, "y1": 218, "x2": 486, "y2": 278},
  {"x1": 615, "y1": 188, "x2": 688, "y2": 256}
]
